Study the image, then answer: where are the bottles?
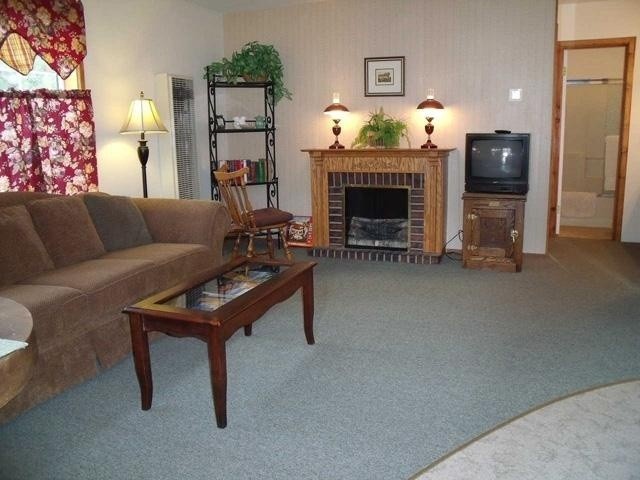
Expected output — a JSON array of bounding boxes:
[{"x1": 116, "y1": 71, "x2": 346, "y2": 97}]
[
  {"x1": 254, "y1": 115, "x2": 264, "y2": 129},
  {"x1": 234, "y1": 117, "x2": 246, "y2": 129},
  {"x1": 216, "y1": 114, "x2": 225, "y2": 130}
]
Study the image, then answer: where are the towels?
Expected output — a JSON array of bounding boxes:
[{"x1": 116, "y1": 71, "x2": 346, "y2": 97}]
[
  {"x1": 562, "y1": 189, "x2": 598, "y2": 220},
  {"x1": 604, "y1": 135, "x2": 619, "y2": 192}
]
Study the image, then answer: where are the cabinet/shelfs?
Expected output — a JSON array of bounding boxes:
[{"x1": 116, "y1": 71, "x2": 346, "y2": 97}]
[
  {"x1": 460, "y1": 190, "x2": 526, "y2": 271},
  {"x1": 201, "y1": 76, "x2": 282, "y2": 247}
]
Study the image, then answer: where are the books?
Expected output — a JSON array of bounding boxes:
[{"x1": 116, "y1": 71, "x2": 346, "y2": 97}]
[
  {"x1": 286, "y1": 215, "x2": 314, "y2": 249},
  {"x1": 212, "y1": 160, "x2": 272, "y2": 185}
]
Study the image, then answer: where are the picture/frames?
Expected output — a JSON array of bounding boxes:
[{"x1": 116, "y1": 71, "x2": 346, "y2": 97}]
[{"x1": 364, "y1": 55, "x2": 406, "y2": 98}]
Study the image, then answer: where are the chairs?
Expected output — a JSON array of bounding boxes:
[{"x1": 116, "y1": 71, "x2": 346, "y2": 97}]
[{"x1": 213, "y1": 164, "x2": 291, "y2": 259}]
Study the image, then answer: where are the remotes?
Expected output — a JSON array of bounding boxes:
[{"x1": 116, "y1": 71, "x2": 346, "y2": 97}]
[{"x1": 495, "y1": 130, "x2": 511, "y2": 134}]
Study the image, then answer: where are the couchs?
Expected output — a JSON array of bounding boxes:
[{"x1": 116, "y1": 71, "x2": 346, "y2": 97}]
[{"x1": 0, "y1": 189, "x2": 230, "y2": 424}]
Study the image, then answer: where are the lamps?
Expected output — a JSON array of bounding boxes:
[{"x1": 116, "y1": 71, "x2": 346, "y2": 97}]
[
  {"x1": 324, "y1": 91, "x2": 349, "y2": 148},
  {"x1": 416, "y1": 86, "x2": 443, "y2": 149},
  {"x1": 121, "y1": 90, "x2": 169, "y2": 196}
]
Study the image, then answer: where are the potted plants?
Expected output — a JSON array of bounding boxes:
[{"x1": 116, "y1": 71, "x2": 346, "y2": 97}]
[
  {"x1": 201, "y1": 37, "x2": 294, "y2": 109},
  {"x1": 350, "y1": 107, "x2": 411, "y2": 149}
]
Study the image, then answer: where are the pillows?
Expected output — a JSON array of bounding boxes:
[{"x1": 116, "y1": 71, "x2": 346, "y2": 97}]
[
  {"x1": 1, "y1": 203, "x2": 52, "y2": 285},
  {"x1": 84, "y1": 191, "x2": 153, "y2": 249},
  {"x1": 28, "y1": 194, "x2": 99, "y2": 266}
]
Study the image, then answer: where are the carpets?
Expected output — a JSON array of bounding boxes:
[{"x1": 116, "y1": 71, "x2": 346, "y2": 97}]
[{"x1": 407, "y1": 378, "x2": 639, "y2": 478}]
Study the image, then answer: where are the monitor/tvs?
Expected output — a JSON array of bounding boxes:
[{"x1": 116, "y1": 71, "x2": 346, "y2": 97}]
[{"x1": 464, "y1": 133, "x2": 530, "y2": 195}]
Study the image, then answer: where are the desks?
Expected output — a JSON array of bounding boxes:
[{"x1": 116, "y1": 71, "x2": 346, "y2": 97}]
[{"x1": 1, "y1": 294, "x2": 38, "y2": 411}]
